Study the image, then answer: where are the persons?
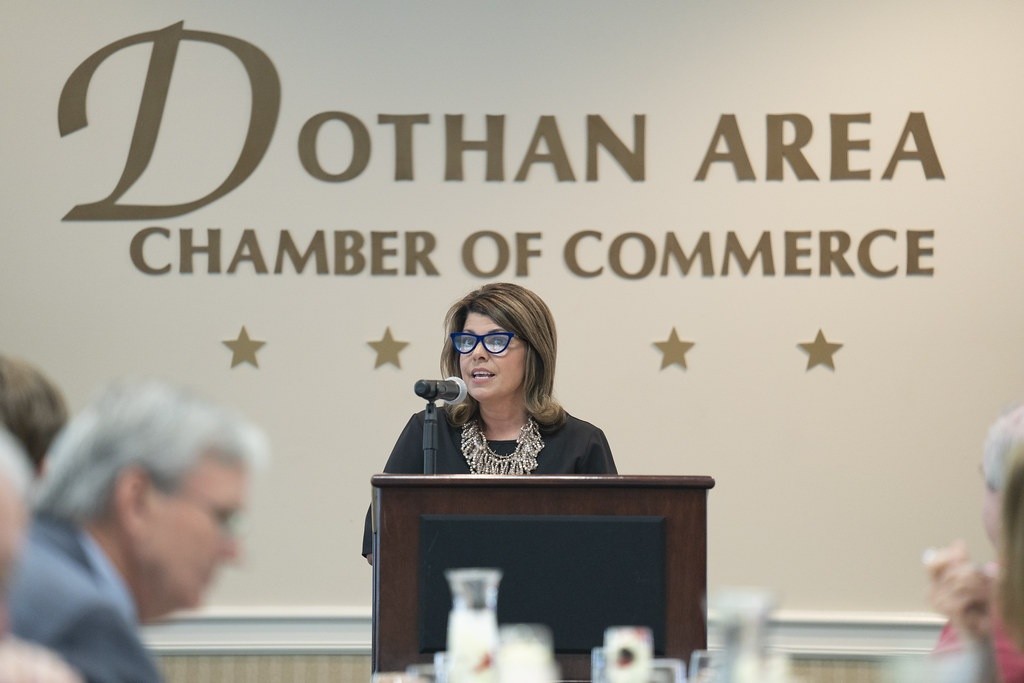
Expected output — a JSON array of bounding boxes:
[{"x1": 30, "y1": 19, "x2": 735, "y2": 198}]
[
  {"x1": 923, "y1": 404, "x2": 1023, "y2": 680},
  {"x1": 1, "y1": 349, "x2": 265, "y2": 683},
  {"x1": 360, "y1": 283, "x2": 621, "y2": 568}
]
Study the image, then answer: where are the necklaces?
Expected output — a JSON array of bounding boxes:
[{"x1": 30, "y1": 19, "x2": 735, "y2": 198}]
[{"x1": 459, "y1": 412, "x2": 545, "y2": 476}]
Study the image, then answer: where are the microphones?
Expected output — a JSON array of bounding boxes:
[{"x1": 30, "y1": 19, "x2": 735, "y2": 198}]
[{"x1": 414, "y1": 376, "x2": 467, "y2": 406}]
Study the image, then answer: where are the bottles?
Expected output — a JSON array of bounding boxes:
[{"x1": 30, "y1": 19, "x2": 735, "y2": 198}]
[{"x1": 379, "y1": 564, "x2": 790, "y2": 683}]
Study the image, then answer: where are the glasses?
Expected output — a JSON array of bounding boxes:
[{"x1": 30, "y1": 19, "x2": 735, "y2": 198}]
[{"x1": 450, "y1": 332, "x2": 516, "y2": 354}]
